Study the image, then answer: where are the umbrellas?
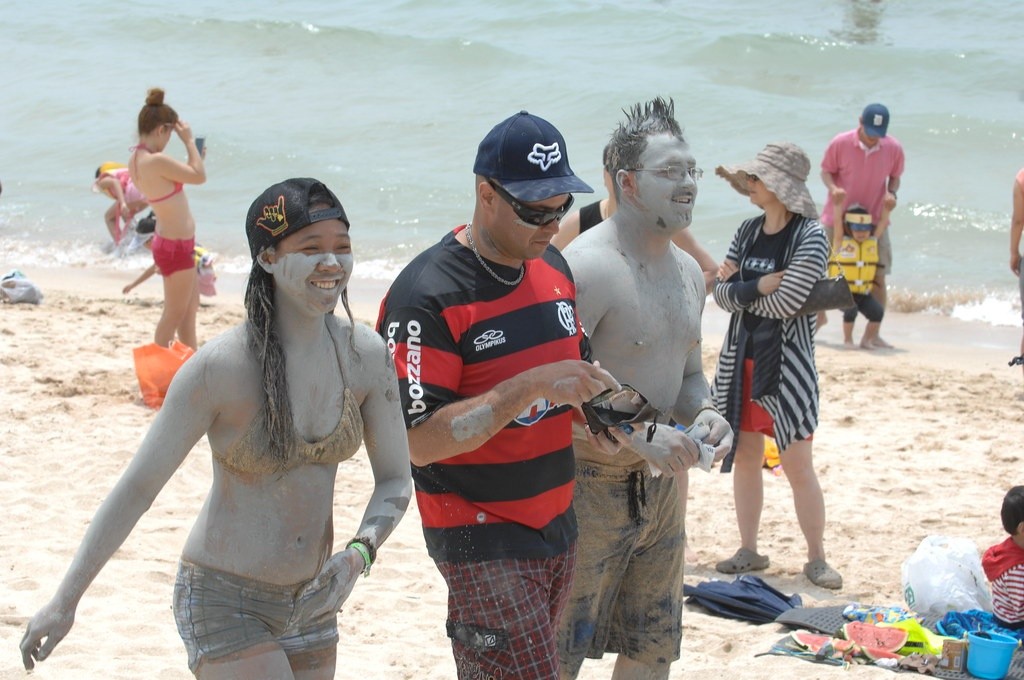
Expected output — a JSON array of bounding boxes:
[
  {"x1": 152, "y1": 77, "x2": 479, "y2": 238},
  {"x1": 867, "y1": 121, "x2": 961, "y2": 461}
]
[{"x1": 684, "y1": 574, "x2": 803, "y2": 625}]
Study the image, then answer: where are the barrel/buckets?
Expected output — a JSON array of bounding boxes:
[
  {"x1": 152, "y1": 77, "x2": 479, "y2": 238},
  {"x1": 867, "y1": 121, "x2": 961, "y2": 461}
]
[{"x1": 963, "y1": 630, "x2": 1022, "y2": 680}]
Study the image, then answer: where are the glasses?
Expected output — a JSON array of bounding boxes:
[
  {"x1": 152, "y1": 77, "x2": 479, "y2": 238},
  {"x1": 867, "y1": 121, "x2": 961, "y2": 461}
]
[
  {"x1": 746, "y1": 175, "x2": 758, "y2": 181},
  {"x1": 632, "y1": 166, "x2": 704, "y2": 182},
  {"x1": 486, "y1": 178, "x2": 575, "y2": 227}
]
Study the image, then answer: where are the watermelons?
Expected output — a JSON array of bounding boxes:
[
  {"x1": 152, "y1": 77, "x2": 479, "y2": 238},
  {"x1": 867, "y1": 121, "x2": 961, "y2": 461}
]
[{"x1": 791, "y1": 620, "x2": 909, "y2": 664}]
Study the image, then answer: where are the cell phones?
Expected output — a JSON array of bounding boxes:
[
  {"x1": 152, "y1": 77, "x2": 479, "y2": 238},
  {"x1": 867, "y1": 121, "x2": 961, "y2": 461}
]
[{"x1": 195, "y1": 137, "x2": 205, "y2": 154}]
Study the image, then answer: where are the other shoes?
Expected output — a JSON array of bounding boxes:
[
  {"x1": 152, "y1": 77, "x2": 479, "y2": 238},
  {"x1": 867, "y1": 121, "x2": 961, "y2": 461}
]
[{"x1": 1008, "y1": 352, "x2": 1024, "y2": 366}]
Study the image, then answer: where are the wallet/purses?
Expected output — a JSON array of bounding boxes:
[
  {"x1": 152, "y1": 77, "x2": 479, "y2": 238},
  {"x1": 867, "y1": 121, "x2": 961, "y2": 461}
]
[{"x1": 583, "y1": 383, "x2": 661, "y2": 443}]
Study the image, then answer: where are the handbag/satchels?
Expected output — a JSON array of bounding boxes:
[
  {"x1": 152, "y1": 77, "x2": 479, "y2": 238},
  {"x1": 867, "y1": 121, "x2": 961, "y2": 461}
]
[
  {"x1": 132, "y1": 342, "x2": 194, "y2": 407},
  {"x1": 901, "y1": 534, "x2": 993, "y2": 627},
  {"x1": 791, "y1": 218, "x2": 856, "y2": 319}
]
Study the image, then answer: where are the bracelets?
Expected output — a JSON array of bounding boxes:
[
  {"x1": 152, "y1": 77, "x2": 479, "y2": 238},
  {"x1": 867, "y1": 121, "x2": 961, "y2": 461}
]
[
  {"x1": 344, "y1": 537, "x2": 376, "y2": 577},
  {"x1": 691, "y1": 404, "x2": 721, "y2": 422},
  {"x1": 888, "y1": 190, "x2": 897, "y2": 199}
]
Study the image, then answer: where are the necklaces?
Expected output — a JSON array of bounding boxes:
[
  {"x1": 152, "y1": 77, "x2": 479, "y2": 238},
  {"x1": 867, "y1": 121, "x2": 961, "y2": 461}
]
[{"x1": 465, "y1": 221, "x2": 525, "y2": 285}]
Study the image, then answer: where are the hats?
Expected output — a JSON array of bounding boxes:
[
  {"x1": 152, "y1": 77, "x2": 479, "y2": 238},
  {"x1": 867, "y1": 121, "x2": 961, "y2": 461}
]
[
  {"x1": 845, "y1": 214, "x2": 874, "y2": 231},
  {"x1": 128, "y1": 232, "x2": 153, "y2": 252},
  {"x1": 475, "y1": 111, "x2": 595, "y2": 202},
  {"x1": 862, "y1": 103, "x2": 890, "y2": 138},
  {"x1": 715, "y1": 145, "x2": 820, "y2": 220},
  {"x1": 246, "y1": 177, "x2": 350, "y2": 258}
]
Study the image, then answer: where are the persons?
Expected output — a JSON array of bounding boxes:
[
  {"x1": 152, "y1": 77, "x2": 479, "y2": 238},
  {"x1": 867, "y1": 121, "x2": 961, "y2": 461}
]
[
  {"x1": 982, "y1": 486, "x2": 1024, "y2": 631},
  {"x1": 1008, "y1": 167, "x2": 1024, "y2": 367},
  {"x1": 556, "y1": 95, "x2": 735, "y2": 680},
  {"x1": 827, "y1": 199, "x2": 890, "y2": 349},
  {"x1": 373, "y1": 110, "x2": 645, "y2": 680},
  {"x1": 91, "y1": 162, "x2": 150, "y2": 245},
  {"x1": 814, "y1": 103, "x2": 904, "y2": 348},
  {"x1": 122, "y1": 209, "x2": 217, "y2": 297},
  {"x1": 549, "y1": 164, "x2": 720, "y2": 296},
  {"x1": 128, "y1": 88, "x2": 207, "y2": 352},
  {"x1": 710, "y1": 141, "x2": 843, "y2": 589},
  {"x1": 20, "y1": 176, "x2": 412, "y2": 680}
]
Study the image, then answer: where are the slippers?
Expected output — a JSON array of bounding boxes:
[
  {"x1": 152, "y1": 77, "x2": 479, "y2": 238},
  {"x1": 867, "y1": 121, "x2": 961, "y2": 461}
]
[
  {"x1": 804, "y1": 561, "x2": 842, "y2": 589},
  {"x1": 716, "y1": 548, "x2": 770, "y2": 574}
]
